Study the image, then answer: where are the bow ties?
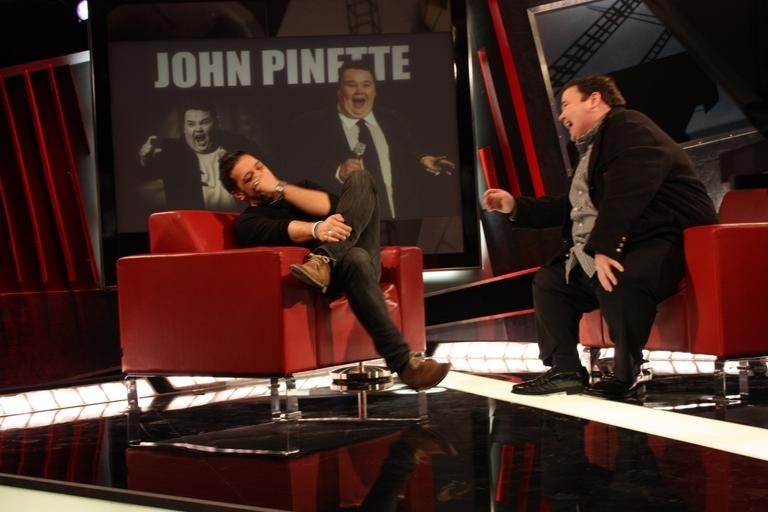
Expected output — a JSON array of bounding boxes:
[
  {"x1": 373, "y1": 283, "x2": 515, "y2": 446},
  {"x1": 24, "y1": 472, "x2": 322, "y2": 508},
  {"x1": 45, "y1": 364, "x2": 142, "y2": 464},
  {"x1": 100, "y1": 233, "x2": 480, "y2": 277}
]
[{"x1": 576, "y1": 132, "x2": 595, "y2": 155}]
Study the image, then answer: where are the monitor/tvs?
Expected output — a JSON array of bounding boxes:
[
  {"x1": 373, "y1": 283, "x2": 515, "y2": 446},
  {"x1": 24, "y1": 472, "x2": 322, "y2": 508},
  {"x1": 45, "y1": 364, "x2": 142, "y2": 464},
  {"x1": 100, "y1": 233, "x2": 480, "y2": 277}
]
[{"x1": 88, "y1": 0, "x2": 482, "y2": 289}]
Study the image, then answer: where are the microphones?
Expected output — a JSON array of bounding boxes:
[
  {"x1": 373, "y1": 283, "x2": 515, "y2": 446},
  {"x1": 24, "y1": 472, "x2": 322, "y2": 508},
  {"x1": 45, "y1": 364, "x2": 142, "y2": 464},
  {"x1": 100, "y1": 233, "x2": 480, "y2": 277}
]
[{"x1": 350, "y1": 142, "x2": 367, "y2": 160}]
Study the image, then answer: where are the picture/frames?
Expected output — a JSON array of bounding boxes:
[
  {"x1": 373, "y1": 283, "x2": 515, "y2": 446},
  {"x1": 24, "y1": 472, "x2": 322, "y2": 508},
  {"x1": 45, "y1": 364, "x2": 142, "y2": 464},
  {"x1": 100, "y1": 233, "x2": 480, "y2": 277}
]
[{"x1": 526, "y1": 0, "x2": 757, "y2": 180}]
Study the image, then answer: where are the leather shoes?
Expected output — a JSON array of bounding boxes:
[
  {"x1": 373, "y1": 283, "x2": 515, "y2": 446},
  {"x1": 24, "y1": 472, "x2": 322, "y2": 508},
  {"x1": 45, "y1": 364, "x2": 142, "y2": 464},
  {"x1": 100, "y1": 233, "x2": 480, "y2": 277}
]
[
  {"x1": 513, "y1": 363, "x2": 647, "y2": 405},
  {"x1": 288, "y1": 253, "x2": 336, "y2": 298},
  {"x1": 398, "y1": 351, "x2": 451, "y2": 392}
]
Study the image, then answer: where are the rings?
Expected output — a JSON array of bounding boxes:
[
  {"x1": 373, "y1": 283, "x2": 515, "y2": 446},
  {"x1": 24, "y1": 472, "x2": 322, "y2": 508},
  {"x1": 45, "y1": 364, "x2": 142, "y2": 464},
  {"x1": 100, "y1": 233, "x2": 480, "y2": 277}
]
[
  {"x1": 254, "y1": 181, "x2": 259, "y2": 185},
  {"x1": 327, "y1": 229, "x2": 333, "y2": 235}
]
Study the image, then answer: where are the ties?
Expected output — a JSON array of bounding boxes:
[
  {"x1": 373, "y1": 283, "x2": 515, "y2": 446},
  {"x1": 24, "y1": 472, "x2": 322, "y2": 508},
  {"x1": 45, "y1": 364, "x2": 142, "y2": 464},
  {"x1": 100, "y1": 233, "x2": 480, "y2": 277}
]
[{"x1": 356, "y1": 119, "x2": 392, "y2": 221}]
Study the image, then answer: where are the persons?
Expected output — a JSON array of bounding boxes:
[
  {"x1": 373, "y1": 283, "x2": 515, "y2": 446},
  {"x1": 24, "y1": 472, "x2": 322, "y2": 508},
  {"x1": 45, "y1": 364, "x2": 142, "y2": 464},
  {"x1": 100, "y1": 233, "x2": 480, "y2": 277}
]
[
  {"x1": 484, "y1": 76, "x2": 723, "y2": 397},
  {"x1": 140, "y1": 101, "x2": 275, "y2": 211},
  {"x1": 512, "y1": 406, "x2": 684, "y2": 512},
  {"x1": 307, "y1": 60, "x2": 455, "y2": 188},
  {"x1": 352, "y1": 425, "x2": 453, "y2": 511},
  {"x1": 219, "y1": 150, "x2": 452, "y2": 391}
]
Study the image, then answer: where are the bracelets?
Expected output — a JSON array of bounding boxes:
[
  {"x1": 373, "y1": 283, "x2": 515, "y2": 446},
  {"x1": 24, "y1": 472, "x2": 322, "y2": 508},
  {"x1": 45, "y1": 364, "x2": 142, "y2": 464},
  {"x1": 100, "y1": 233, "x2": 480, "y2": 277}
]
[{"x1": 311, "y1": 221, "x2": 324, "y2": 242}]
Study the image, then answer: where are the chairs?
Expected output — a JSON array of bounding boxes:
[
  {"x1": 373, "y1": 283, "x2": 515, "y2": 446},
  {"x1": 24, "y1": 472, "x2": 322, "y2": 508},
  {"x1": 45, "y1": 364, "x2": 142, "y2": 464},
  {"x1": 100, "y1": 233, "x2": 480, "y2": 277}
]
[
  {"x1": 122, "y1": 416, "x2": 439, "y2": 510},
  {"x1": 115, "y1": 207, "x2": 429, "y2": 417},
  {"x1": 572, "y1": 187, "x2": 767, "y2": 391}
]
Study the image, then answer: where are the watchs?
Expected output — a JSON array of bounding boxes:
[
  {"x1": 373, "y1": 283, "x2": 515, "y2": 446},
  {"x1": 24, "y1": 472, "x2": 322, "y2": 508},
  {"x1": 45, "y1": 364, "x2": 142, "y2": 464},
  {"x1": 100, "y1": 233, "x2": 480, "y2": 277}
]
[{"x1": 273, "y1": 181, "x2": 287, "y2": 203}]
